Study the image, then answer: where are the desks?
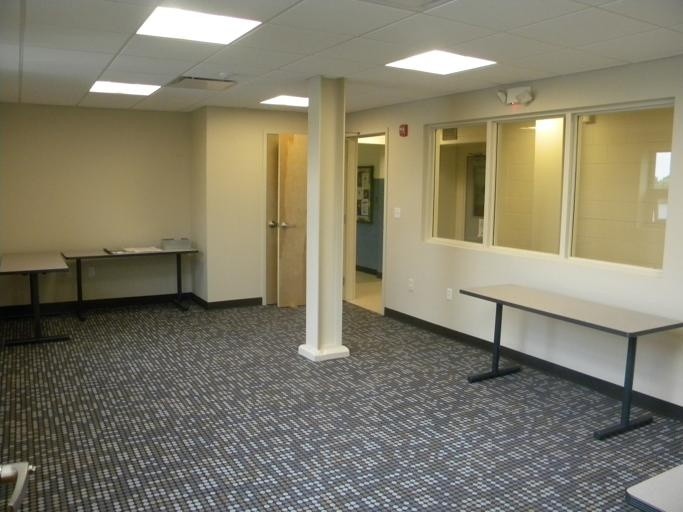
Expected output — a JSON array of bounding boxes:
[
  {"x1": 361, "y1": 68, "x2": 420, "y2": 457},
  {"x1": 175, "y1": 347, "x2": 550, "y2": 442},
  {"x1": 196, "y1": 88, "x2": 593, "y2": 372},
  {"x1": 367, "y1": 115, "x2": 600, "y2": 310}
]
[
  {"x1": 61, "y1": 247, "x2": 199, "y2": 319},
  {"x1": 459, "y1": 284, "x2": 683, "y2": 439},
  {"x1": 626, "y1": 465, "x2": 683, "y2": 512},
  {"x1": 0, "y1": 251, "x2": 69, "y2": 347}
]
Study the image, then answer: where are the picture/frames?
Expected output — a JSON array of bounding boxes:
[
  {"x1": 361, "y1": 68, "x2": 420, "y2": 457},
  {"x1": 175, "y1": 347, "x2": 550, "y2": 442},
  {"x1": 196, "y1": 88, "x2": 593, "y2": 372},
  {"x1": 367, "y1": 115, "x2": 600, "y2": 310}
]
[{"x1": 357, "y1": 166, "x2": 375, "y2": 223}]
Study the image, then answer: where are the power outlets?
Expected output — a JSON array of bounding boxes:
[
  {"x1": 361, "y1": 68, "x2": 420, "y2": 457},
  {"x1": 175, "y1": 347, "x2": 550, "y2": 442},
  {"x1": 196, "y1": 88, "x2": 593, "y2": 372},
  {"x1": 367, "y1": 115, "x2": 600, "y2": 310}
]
[{"x1": 447, "y1": 288, "x2": 452, "y2": 300}]
